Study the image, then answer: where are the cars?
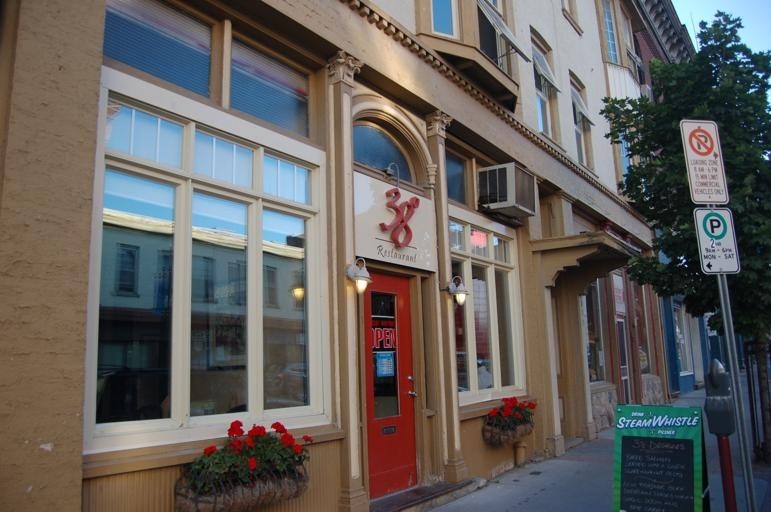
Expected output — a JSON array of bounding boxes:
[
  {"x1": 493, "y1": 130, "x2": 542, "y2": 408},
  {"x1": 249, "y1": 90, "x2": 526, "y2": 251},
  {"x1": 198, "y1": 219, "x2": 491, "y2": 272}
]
[{"x1": 457, "y1": 351, "x2": 491, "y2": 390}]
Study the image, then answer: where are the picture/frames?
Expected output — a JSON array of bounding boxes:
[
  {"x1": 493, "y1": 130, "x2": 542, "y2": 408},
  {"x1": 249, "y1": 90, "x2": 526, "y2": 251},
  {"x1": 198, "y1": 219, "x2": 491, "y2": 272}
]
[{"x1": 205, "y1": 276, "x2": 247, "y2": 371}]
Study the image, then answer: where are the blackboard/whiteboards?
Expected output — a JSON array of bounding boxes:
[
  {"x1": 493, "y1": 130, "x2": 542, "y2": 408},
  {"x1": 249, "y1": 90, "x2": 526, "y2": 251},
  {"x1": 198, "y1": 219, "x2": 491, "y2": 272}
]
[{"x1": 612, "y1": 402, "x2": 703, "y2": 512}]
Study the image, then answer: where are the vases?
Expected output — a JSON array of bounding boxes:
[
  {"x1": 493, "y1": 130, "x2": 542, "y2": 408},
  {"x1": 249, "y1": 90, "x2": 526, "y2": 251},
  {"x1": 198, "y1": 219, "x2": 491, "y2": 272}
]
[
  {"x1": 483, "y1": 421, "x2": 534, "y2": 448},
  {"x1": 174, "y1": 469, "x2": 310, "y2": 512}
]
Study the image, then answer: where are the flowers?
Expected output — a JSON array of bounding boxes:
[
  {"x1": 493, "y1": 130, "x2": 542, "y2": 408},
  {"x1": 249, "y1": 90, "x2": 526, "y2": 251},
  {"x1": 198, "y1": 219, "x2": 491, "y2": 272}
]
[
  {"x1": 182, "y1": 416, "x2": 313, "y2": 500},
  {"x1": 483, "y1": 394, "x2": 537, "y2": 432}
]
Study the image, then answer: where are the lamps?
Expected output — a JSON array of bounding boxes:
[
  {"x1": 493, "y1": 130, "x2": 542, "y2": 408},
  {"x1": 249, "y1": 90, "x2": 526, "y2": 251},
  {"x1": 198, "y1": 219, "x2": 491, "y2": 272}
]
[
  {"x1": 289, "y1": 282, "x2": 305, "y2": 307},
  {"x1": 440, "y1": 274, "x2": 469, "y2": 308},
  {"x1": 346, "y1": 254, "x2": 373, "y2": 296}
]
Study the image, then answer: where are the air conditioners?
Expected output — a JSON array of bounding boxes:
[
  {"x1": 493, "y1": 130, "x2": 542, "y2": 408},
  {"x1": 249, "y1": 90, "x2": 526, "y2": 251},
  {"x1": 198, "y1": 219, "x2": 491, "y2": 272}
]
[{"x1": 476, "y1": 161, "x2": 538, "y2": 220}]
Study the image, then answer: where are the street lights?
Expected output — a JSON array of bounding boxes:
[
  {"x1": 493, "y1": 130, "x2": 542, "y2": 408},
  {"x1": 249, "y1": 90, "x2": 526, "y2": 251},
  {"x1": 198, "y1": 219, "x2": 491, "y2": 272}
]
[{"x1": 288, "y1": 283, "x2": 308, "y2": 405}]
